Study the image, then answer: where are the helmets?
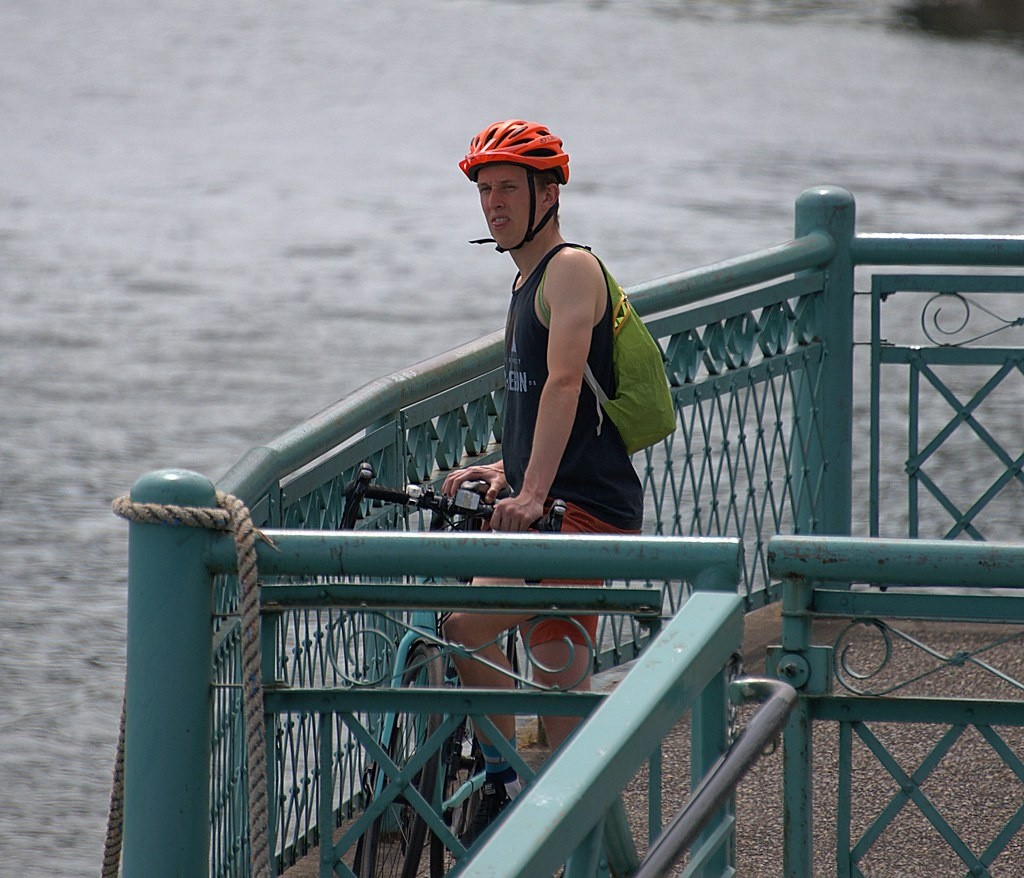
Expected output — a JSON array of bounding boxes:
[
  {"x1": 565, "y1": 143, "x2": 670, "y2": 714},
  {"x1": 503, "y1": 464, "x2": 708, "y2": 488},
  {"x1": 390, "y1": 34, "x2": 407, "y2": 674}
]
[{"x1": 459, "y1": 120, "x2": 569, "y2": 185}]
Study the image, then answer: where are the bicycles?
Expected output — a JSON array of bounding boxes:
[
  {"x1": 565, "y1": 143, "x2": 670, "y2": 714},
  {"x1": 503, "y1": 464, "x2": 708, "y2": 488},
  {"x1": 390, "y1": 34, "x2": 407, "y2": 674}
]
[{"x1": 338, "y1": 460, "x2": 569, "y2": 878}]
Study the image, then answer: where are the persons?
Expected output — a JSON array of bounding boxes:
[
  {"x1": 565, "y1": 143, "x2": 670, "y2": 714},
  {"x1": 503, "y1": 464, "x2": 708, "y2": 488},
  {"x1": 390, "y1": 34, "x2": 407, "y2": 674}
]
[{"x1": 443, "y1": 120, "x2": 645, "y2": 849}]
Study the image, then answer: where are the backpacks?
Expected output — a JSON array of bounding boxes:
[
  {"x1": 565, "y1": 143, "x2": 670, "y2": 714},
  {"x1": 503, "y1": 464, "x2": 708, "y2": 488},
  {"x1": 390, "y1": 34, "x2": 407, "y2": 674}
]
[{"x1": 537, "y1": 250, "x2": 678, "y2": 455}]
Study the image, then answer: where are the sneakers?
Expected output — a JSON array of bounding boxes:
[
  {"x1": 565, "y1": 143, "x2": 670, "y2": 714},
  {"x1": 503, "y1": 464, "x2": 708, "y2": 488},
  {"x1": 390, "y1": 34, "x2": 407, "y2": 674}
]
[{"x1": 450, "y1": 782, "x2": 512, "y2": 857}]
